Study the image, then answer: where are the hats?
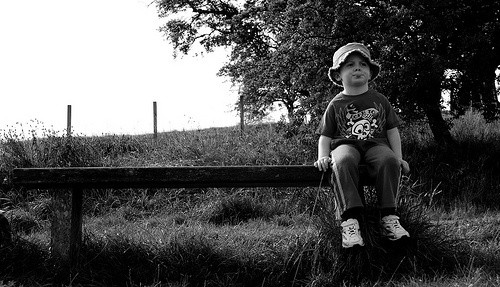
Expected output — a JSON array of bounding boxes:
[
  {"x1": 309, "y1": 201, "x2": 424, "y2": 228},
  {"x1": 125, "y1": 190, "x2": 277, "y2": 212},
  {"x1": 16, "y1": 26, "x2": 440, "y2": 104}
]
[{"x1": 327, "y1": 42, "x2": 381, "y2": 86}]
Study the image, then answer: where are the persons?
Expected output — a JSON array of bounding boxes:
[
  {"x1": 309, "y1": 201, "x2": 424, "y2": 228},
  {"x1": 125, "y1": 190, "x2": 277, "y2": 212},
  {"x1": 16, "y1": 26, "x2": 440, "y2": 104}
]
[{"x1": 313, "y1": 42, "x2": 412, "y2": 251}]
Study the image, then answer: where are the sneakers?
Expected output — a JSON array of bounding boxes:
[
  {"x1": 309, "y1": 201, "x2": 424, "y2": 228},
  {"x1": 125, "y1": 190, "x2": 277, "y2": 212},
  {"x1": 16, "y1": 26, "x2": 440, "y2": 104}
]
[
  {"x1": 379, "y1": 215, "x2": 410, "y2": 241},
  {"x1": 340, "y1": 219, "x2": 365, "y2": 249}
]
